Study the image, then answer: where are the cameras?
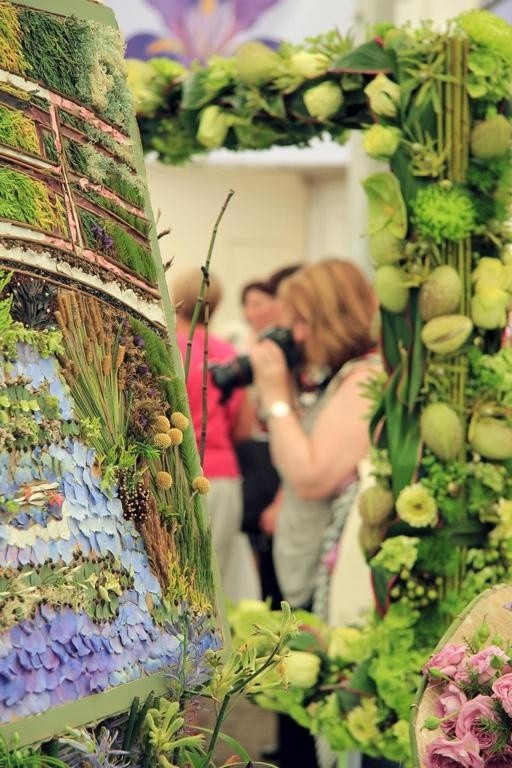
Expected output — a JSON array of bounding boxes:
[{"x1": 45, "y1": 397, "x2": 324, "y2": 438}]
[{"x1": 205, "y1": 317, "x2": 304, "y2": 406}]
